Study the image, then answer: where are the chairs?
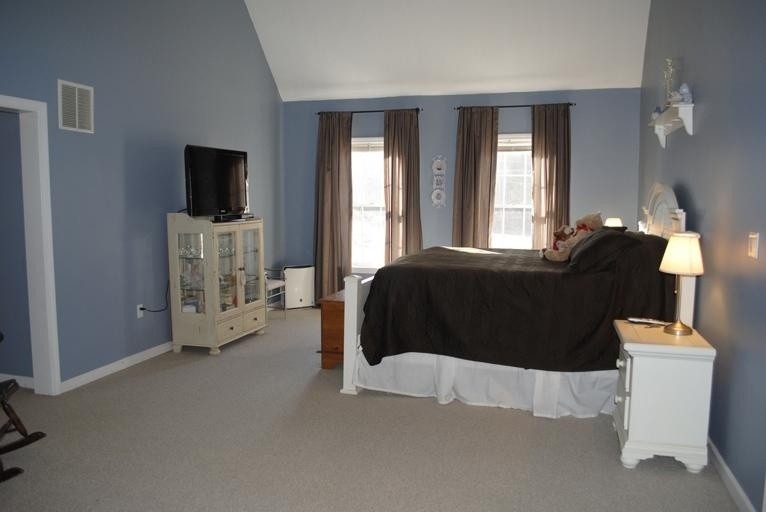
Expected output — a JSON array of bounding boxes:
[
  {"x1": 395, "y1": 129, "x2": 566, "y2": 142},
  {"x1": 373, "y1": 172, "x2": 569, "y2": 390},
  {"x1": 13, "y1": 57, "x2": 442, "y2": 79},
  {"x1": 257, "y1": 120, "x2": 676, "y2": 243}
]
[
  {"x1": 0, "y1": 333, "x2": 47, "y2": 485},
  {"x1": 264, "y1": 266, "x2": 288, "y2": 325}
]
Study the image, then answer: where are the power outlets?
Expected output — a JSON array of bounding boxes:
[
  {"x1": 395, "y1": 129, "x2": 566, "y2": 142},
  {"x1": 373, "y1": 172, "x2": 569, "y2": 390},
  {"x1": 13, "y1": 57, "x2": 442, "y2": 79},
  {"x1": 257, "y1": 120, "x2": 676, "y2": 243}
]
[{"x1": 136, "y1": 303, "x2": 144, "y2": 319}]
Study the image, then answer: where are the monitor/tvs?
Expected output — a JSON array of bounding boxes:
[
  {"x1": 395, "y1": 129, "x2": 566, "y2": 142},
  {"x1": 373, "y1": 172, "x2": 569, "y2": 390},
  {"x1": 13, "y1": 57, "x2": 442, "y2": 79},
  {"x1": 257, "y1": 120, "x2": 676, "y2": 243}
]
[{"x1": 184, "y1": 144, "x2": 247, "y2": 223}]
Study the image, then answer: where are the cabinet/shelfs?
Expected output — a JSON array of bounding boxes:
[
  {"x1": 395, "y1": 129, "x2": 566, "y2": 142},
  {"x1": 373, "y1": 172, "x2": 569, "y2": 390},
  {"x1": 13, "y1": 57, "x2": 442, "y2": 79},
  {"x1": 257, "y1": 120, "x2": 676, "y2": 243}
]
[{"x1": 166, "y1": 210, "x2": 270, "y2": 359}]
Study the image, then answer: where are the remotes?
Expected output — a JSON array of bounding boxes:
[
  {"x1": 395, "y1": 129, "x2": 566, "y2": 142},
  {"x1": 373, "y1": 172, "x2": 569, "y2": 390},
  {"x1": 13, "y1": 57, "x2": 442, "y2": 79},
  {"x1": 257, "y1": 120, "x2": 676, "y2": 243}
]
[{"x1": 627, "y1": 317, "x2": 667, "y2": 325}]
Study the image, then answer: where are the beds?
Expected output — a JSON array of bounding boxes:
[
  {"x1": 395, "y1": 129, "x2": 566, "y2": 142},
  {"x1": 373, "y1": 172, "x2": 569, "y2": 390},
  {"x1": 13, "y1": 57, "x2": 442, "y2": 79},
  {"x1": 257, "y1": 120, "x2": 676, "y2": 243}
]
[{"x1": 341, "y1": 180, "x2": 687, "y2": 419}]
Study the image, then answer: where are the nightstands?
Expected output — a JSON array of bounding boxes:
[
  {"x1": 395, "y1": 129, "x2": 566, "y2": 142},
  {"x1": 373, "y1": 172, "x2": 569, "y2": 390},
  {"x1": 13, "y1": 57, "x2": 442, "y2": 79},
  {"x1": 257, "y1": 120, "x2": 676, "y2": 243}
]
[{"x1": 613, "y1": 315, "x2": 718, "y2": 473}]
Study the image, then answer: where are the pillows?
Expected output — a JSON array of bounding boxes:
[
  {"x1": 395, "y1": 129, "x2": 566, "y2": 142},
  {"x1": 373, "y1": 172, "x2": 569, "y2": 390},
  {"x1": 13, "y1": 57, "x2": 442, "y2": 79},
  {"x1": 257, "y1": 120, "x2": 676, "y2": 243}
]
[
  {"x1": 600, "y1": 224, "x2": 626, "y2": 231},
  {"x1": 568, "y1": 229, "x2": 642, "y2": 274}
]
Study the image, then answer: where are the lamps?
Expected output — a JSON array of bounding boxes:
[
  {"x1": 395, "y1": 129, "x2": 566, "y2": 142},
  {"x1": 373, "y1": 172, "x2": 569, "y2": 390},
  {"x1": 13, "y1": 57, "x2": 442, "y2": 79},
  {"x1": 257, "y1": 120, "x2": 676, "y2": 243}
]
[{"x1": 656, "y1": 230, "x2": 704, "y2": 339}]
